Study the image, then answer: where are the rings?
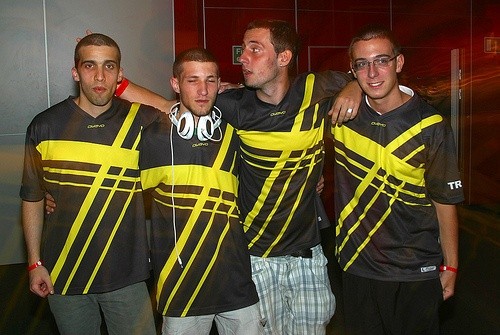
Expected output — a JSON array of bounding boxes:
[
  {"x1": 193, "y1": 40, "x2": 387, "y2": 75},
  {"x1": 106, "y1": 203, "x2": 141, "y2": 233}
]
[{"x1": 347, "y1": 107, "x2": 353, "y2": 114}]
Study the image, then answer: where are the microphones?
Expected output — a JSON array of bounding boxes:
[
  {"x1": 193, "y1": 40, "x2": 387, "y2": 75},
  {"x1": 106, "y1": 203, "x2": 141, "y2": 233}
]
[{"x1": 201, "y1": 126, "x2": 222, "y2": 141}]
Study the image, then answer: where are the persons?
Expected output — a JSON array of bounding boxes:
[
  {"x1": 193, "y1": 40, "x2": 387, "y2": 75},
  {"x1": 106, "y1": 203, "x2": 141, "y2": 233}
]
[
  {"x1": 45, "y1": 46, "x2": 326, "y2": 335},
  {"x1": 20, "y1": 33, "x2": 168, "y2": 335},
  {"x1": 75, "y1": 28, "x2": 363, "y2": 335},
  {"x1": 216, "y1": 19, "x2": 465, "y2": 335}
]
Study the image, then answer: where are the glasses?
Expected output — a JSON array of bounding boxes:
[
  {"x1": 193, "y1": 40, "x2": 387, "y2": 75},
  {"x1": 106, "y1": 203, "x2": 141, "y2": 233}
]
[{"x1": 351, "y1": 53, "x2": 398, "y2": 72}]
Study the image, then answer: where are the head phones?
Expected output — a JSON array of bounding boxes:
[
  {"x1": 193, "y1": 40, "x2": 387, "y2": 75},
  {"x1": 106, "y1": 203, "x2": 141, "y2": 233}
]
[{"x1": 169, "y1": 102, "x2": 222, "y2": 141}]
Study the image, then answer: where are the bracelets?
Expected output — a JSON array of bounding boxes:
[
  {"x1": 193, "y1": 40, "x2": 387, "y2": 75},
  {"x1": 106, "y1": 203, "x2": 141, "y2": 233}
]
[
  {"x1": 439, "y1": 264, "x2": 458, "y2": 274},
  {"x1": 114, "y1": 78, "x2": 131, "y2": 97},
  {"x1": 27, "y1": 259, "x2": 44, "y2": 272}
]
